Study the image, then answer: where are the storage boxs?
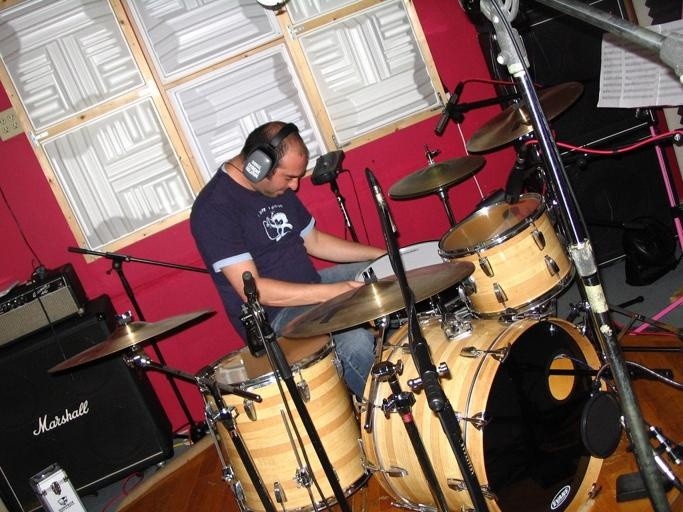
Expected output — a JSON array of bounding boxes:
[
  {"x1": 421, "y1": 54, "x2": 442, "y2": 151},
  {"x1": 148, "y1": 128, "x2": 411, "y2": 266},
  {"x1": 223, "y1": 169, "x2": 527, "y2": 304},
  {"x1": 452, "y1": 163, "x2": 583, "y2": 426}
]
[
  {"x1": 0, "y1": 275, "x2": 81, "y2": 347},
  {"x1": 27, "y1": 465, "x2": 87, "y2": 512}
]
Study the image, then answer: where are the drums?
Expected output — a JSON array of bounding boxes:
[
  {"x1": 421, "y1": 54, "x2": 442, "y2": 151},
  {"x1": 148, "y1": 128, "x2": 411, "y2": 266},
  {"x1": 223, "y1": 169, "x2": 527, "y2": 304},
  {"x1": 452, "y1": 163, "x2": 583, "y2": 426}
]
[
  {"x1": 438, "y1": 194, "x2": 578, "y2": 321},
  {"x1": 194, "y1": 333, "x2": 371, "y2": 511},
  {"x1": 358, "y1": 310, "x2": 609, "y2": 512},
  {"x1": 354, "y1": 240, "x2": 474, "y2": 330}
]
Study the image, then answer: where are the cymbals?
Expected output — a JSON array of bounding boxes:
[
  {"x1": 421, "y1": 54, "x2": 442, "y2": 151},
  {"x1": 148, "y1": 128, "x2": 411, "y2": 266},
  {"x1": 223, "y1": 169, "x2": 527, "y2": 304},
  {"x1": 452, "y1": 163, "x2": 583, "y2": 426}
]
[
  {"x1": 388, "y1": 152, "x2": 486, "y2": 201},
  {"x1": 45, "y1": 310, "x2": 217, "y2": 375},
  {"x1": 280, "y1": 262, "x2": 475, "y2": 339},
  {"x1": 465, "y1": 80, "x2": 583, "y2": 154}
]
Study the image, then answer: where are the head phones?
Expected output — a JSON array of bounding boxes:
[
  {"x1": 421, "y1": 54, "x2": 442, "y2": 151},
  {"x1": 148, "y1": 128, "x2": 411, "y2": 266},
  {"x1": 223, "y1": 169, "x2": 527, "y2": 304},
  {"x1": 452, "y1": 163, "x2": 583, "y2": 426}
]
[{"x1": 241, "y1": 123, "x2": 299, "y2": 185}]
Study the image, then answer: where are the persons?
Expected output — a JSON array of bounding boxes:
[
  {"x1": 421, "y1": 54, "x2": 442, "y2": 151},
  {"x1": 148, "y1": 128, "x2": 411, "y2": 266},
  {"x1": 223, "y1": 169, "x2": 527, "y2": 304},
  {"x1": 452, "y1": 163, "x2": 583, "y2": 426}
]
[{"x1": 188, "y1": 120, "x2": 390, "y2": 408}]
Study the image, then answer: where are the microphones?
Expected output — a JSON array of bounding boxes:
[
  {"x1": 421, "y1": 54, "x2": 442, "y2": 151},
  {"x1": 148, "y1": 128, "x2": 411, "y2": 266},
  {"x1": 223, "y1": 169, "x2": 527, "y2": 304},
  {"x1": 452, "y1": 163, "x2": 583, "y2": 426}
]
[
  {"x1": 364, "y1": 167, "x2": 400, "y2": 240},
  {"x1": 242, "y1": 271, "x2": 256, "y2": 302},
  {"x1": 433, "y1": 82, "x2": 463, "y2": 134}
]
